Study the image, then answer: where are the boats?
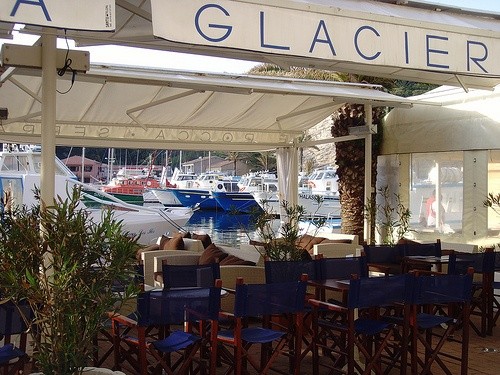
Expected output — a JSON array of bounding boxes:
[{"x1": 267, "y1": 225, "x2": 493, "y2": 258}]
[{"x1": 0, "y1": 142, "x2": 464, "y2": 269}]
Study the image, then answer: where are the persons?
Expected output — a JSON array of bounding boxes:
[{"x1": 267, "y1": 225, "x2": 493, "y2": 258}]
[
  {"x1": 425, "y1": 189, "x2": 438, "y2": 220},
  {"x1": 428, "y1": 193, "x2": 445, "y2": 228}
]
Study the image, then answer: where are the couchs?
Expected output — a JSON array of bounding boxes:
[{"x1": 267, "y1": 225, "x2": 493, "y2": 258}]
[
  {"x1": 134, "y1": 234, "x2": 266, "y2": 291},
  {"x1": 264, "y1": 233, "x2": 359, "y2": 258}
]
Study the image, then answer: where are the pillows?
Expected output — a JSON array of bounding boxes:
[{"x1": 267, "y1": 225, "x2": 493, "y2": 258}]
[
  {"x1": 160, "y1": 235, "x2": 184, "y2": 250},
  {"x1": 219, "y1": 255, "x2": 256, "y2": 266},
  {"x1": 198, "y1": 243, "x2": 228, "y2": 265},
  {"x1": 193, "y1": 233, "x2": 212, "y2": 249},
  {"x1": 307, "y1": 239, "x2": 353, "y2": 260},
  {"x1": 296, "y1": 234, "x2": 327, "y2": 252},
  {"x1": 172, "y1": 230, "x2": 191, "y2": 238}
]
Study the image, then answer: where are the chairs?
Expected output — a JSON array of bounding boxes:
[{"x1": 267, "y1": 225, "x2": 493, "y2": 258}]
[{"x1": 88, "y1": 237, "x2": 500, "y2": 375}]
